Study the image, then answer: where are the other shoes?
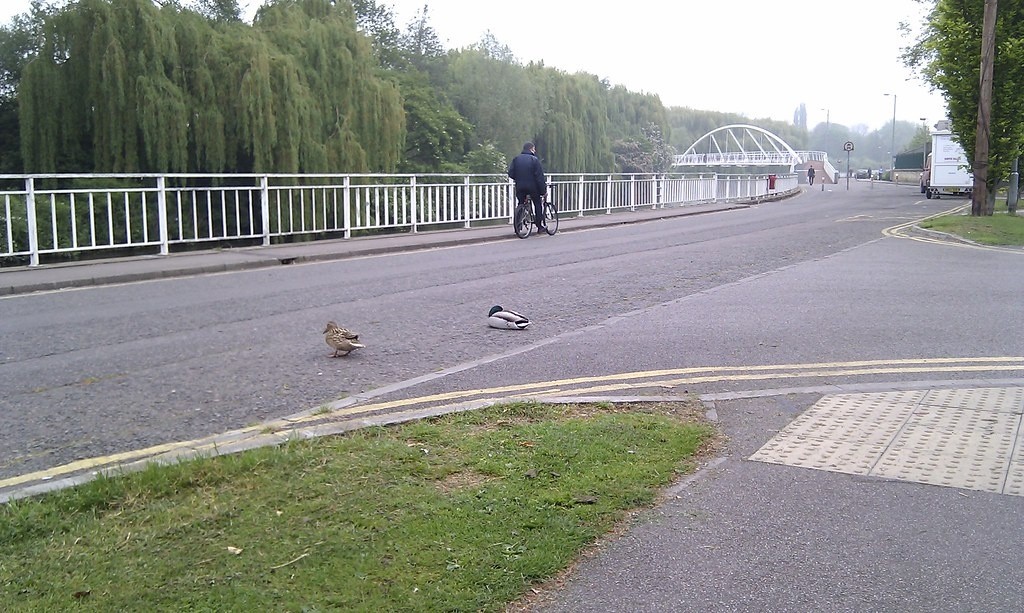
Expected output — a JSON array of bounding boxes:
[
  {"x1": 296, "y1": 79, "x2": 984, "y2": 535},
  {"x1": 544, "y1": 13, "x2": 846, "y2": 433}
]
[
  {"x1": 516, "y1": 232, "x2": 527, "y2": 238},
  {"x1": 537, "y1": 226, "x2": 548, "y2": 233}
]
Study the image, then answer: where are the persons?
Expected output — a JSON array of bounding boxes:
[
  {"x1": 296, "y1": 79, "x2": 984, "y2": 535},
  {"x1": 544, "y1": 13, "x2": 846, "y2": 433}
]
[
  {"x1": 867, "y1": 167, "x2": 872, "y2": 180},
  {"x1": 807, "y1": 165, "x2": 815, "y2": 185},
  {"x1": 508, "y1": 141, "x2": 549, "y2": 234},
  {"x1": 879, "y1": 166, "x2": 883, "y2": 180}
]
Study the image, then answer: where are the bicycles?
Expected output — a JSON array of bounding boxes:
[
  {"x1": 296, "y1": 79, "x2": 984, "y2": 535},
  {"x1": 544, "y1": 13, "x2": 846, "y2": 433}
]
[{"x1": 514, "y1": 184, "x2": 558, "y2": 239}]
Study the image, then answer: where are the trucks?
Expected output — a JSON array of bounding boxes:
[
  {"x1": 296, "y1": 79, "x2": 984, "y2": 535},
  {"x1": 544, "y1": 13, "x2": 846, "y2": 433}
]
[{"x1": 919, "y1": 130, "x2": 974, "y2": 199}]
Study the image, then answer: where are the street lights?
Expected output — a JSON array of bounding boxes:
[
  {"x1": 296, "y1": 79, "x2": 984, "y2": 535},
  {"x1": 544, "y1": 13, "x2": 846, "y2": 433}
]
[
  {"x1": 920, "y1": 117, "x2": 927, "y2": 168},
  {"x1": 884, "y1": 93, "x2": 897, "y2": 182},
  {"x1": 822, "y1": 108, "x2": 829, "y2": 152}
]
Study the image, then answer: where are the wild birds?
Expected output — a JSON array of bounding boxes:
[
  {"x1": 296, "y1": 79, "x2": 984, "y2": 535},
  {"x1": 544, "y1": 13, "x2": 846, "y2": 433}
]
[
  {"x1": 322, "y1": 321, "x2": 366, "y2": 358},
  {"x1": 487, "y1": 305, "x2": 532, "y2": 330}
]
